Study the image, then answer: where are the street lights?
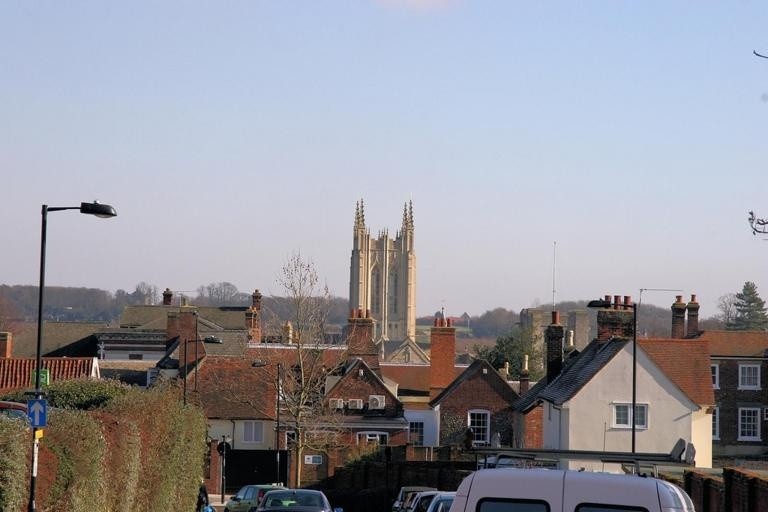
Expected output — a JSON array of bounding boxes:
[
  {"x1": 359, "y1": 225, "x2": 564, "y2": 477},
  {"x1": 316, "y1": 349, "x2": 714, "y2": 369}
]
[
  {"x1": 586, "y1": 299, "x2": 637, "y2": 454},
  {"x1": 27, "y1": 199, "x2": 117, "y2": 511},
  {"x1": 251, "y1": 361, "x2": 280, "y2": 486},
  {"x1": 184, "y1": 335, "x2": 224, "y2": 405}
]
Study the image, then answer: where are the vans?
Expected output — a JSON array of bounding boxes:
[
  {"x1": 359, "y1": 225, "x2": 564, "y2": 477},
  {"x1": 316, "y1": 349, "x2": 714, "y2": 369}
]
[{"x1": 444, "y1": 427, "x2": 697, "y2": 512}]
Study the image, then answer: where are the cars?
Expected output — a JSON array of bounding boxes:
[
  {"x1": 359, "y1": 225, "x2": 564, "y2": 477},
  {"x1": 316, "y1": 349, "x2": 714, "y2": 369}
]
[
  {"x1": 250, "y1": 488, "x2": 343, "y2": 511},
  {"x1": 196, "y1": 485, "x2": 208, "y2": 512},
  {"x1": 390, "y1": 485, "x2": 456, "y2": 512}
]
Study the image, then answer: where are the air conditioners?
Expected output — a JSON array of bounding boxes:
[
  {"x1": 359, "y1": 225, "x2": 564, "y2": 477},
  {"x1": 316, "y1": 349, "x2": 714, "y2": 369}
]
[{"x1": 326, "y1": 394, "x2": 385, "y2": 413}]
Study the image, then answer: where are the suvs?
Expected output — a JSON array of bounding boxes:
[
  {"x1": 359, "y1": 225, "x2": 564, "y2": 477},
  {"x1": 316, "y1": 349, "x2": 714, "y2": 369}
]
[{"x1": 224, "y1": 484, "x2": 289, "y2": 511}]
